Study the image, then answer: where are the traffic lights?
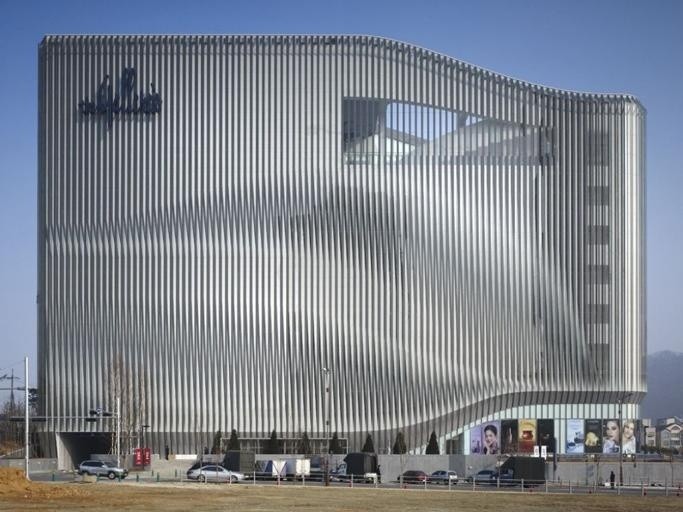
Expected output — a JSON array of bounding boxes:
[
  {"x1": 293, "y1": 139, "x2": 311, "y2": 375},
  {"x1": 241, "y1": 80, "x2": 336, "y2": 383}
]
[{"x1": 86, "y1": 410, "x2": 112, "y2": 422}]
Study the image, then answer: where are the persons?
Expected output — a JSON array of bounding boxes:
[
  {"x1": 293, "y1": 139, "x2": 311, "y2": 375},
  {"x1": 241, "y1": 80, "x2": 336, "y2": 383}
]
[
  {"x1": 609, "y1": 471, "x2": 615, "y2": 490},
  {"x1": 622, "y1": 420, "x2": 637, "y2": 454},
  {"x1": 606, "y1": 420, "x2": 620, "y2": 446},
  {"x1": 482, "y1": 424, "x2": 498, "y2": 454},
  {"x1": 375, "y1": 464, "x2": 382, "y2": 484},
  {"x1": 204, "y1": 446, "x2": 209, "y2": 454},
  {"x1": 165, "y1": 445, "x2": 170, "y2": 460}
]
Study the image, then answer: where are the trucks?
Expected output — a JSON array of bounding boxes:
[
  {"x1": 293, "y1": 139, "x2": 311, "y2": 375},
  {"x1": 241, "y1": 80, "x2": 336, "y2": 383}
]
[
  {"x1": 335, "y1": 451, "x2": 378, "y2": 484},
  {"x1": 221, "y1": 450, "x2": 311, "y2": 481},
  {"x1": 490, "y1": 456, "x2": 546, "y2": 488}
]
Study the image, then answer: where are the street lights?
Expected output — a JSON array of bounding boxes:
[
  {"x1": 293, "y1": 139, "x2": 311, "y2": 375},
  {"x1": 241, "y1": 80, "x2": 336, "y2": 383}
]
[
  {"x1": 320, "y1": 368, "x2": 331, "y2": 478},
  {"x1": 618, "y1": 393, "x2": 635, "y2": 486},
  {"x1": 142, "y1": 424, "x2": 150, "y2": 470}
]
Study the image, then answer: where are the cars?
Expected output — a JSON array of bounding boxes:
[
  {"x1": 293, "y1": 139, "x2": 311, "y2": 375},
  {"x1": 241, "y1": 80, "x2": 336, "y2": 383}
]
[
  {"x1": 467, "y1": 470, "x2": 496, "y2": 484},
  {"x1": 78, "y1": 460, "x2": 128, "y2": 480},
  {"x1": 187, "y1": 464, "x2": 245, "y2": 483},
  {"x1": 397, "y1": 470, "x2": 458, "y2": 485}
]
